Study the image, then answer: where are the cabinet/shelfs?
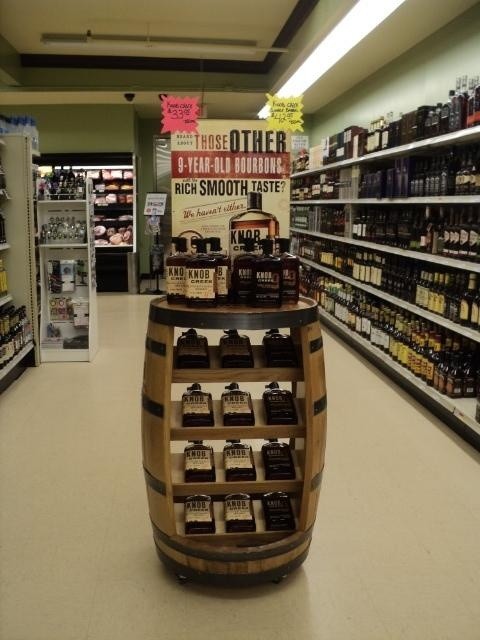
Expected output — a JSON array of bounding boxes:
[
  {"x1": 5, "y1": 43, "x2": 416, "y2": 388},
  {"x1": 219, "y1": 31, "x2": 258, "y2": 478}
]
[
  {"x1": 0, "y1": 130, "x2": 136, "y2": 395},
  {"x1": 140, "y1": 291, "x2": 328, "y2": 590},
  {"x1": 286, "y1": 120, "x2": 480, "y2": 446}
]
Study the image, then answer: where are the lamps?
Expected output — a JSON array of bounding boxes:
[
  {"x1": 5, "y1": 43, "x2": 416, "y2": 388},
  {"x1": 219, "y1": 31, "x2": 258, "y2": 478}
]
[{"x1": 245, "y1": 1, "x2": 405, "y2": 129}]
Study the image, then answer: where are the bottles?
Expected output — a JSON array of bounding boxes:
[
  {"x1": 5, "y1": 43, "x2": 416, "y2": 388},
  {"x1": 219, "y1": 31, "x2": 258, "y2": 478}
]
[
  {"x1": 0, "y1": 116, "x2": 87, "y2": 368},
  {"x1": 165, "y1": 236, "x2": 298, "y2": 533},
  {"x1": 229, "y1": 193, "x2": 279, "y2": 270},
  {"x1": 291, "y1": 74, "x2": 480, "y2": 425}
]
